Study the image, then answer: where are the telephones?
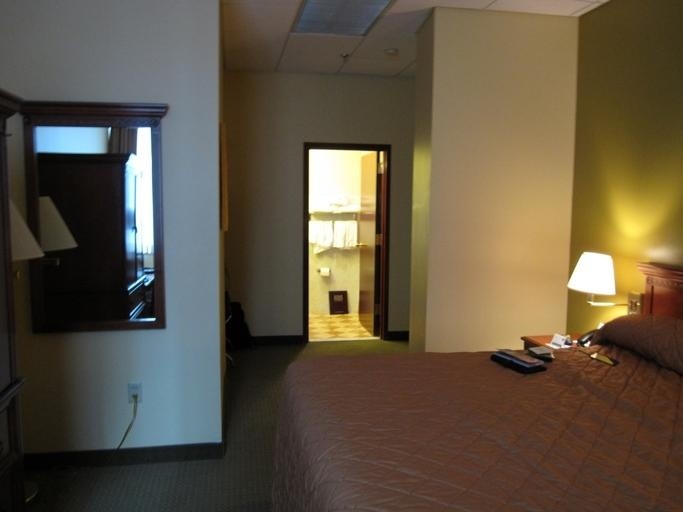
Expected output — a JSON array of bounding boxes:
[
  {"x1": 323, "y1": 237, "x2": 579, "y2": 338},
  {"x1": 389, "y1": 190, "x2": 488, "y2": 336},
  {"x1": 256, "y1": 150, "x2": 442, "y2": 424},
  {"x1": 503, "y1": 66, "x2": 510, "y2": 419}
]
[{"x1": 578, "y1": 327, "x2": 601, "y2": 346}]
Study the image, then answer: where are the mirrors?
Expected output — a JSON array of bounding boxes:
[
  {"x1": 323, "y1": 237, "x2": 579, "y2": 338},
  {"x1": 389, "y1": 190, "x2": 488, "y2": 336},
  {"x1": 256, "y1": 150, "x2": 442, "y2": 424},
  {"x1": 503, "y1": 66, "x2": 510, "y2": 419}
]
[{"x1": 19, "y1": 96, "x2": 162, "y2": 335}]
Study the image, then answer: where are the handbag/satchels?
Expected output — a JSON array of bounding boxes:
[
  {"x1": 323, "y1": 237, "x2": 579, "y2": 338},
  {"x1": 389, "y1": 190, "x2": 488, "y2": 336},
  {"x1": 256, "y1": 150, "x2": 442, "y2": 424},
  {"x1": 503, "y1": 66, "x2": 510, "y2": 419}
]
[{"x1": 491, "y1": 349, "x2": 544, "y2": 373}]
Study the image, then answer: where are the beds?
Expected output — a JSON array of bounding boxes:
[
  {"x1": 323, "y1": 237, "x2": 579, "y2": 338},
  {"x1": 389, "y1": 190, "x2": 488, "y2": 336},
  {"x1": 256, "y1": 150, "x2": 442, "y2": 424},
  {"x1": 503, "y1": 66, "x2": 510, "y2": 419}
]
[{"x1": 277, "y1": 262, "x2": 681, "y2": 511}]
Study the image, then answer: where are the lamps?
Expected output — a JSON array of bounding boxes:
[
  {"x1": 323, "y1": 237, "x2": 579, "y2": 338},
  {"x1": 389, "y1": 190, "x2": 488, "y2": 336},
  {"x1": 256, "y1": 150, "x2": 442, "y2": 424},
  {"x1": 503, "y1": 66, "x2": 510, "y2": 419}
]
[{"x1": 565, "y1": 250, "x2": 643, "y2": 317}]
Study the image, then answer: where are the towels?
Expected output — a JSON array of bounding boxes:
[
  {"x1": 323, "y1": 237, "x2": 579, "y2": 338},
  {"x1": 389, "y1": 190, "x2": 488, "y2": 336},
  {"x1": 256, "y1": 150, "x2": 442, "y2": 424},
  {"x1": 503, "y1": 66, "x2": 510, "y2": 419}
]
[{"x1": 309, "y1": 218, "x2": 357, "y2": 254}]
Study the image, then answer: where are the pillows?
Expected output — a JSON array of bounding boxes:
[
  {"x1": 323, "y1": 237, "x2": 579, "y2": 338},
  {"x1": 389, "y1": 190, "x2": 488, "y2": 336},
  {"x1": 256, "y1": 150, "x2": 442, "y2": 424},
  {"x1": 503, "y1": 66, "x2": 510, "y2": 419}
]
[{"x1": 592, "y1": 307, "x2": 682, "y2": 369}]
